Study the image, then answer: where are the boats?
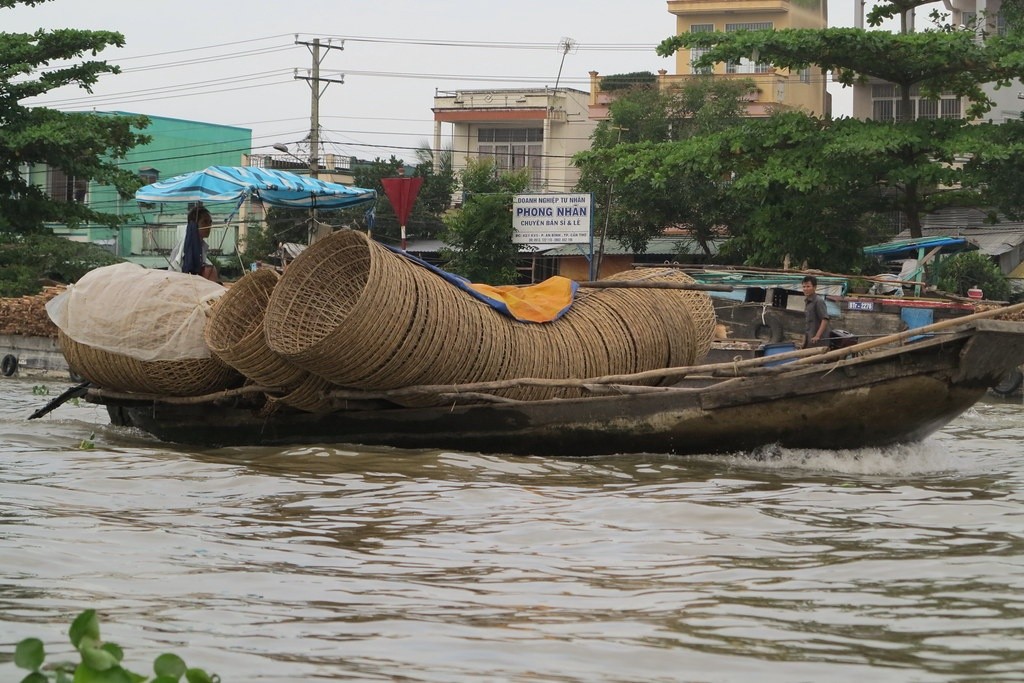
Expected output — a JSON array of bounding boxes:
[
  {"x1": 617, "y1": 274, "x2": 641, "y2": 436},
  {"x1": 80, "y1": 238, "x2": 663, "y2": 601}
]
[{"x1": 83, "y1": 261, "x2": 1023, "y2": 454}]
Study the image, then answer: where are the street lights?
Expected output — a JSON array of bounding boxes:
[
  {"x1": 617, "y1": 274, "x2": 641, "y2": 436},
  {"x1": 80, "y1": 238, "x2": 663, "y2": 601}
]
[{"x1": 272, "y1": 142, "x2": 319, "y2": 222}]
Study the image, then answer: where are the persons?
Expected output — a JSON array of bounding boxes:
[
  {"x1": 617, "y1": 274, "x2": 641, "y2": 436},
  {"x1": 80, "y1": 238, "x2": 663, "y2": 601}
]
[
  {"x1": 799, "y1": 276, "x2": 831, "y2": 351},
  {"x1": 170, "y1": 207, "x2": 220, "y2": 285},
  {"x1": 872, "y1": 252, "x2": 928, "y2": 299}
]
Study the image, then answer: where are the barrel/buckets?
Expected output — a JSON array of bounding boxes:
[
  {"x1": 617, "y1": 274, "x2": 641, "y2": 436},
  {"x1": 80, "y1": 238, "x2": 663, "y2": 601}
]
[{"x1": 761, "y1": 342, "x2": 798, "y2": 368}]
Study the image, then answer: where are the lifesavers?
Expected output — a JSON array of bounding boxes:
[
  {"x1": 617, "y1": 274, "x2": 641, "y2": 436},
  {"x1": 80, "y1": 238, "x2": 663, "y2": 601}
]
[
  {"x1": 991, "y1": 366, "x2": 1022, "y2": 394},
  {"x1": 0, "y1": 353, "x2": 17, "y2": 376},
  {"x1": 745, "y1": 313, "x2": 782, "y2": 345}
]
